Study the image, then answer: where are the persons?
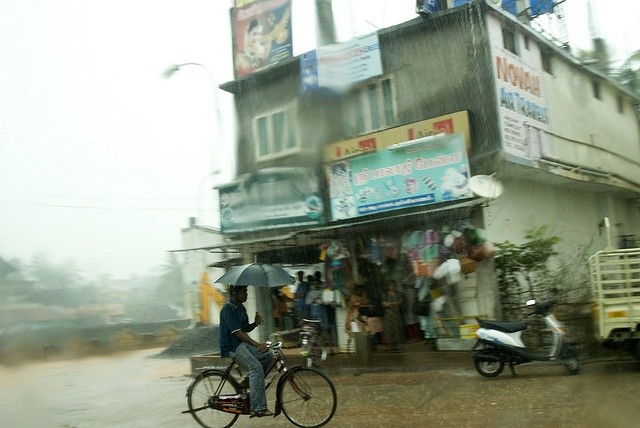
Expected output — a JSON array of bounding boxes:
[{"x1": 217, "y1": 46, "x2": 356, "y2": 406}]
[
  {"x1": 234, "y1": 19, "x2": 269, "y2": 77},
  {"x1": 219, "y1": 285, "x2": 274, "y2": 419},
  {"x1": 381, "y1": 278, "x2": 404, "y2": 352},
  {"x1": 295, "y1": 270, "x2": 327, "y2": 324},
  {"x1": 346, "y1": 284, "x2": 376, "y2": 353}
]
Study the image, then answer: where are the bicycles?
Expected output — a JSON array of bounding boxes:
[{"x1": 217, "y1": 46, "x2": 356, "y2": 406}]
[{"x1": 181, "y1": 342, "x2": 337, "y2": 428}]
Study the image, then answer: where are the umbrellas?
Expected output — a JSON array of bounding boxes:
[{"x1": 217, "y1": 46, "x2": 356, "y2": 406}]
[{"x1": 213, "y1": 254, "x2": 296, "y2": 288}]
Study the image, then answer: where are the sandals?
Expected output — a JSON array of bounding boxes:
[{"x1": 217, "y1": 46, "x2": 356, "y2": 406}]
[{"x1": 249, "y1": 408, "x2": 273, "y2": 418}]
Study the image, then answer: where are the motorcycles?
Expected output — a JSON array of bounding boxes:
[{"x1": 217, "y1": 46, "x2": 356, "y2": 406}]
[{"x1": 470, "y1": 299, "x2": 579, "y2": 378}]
